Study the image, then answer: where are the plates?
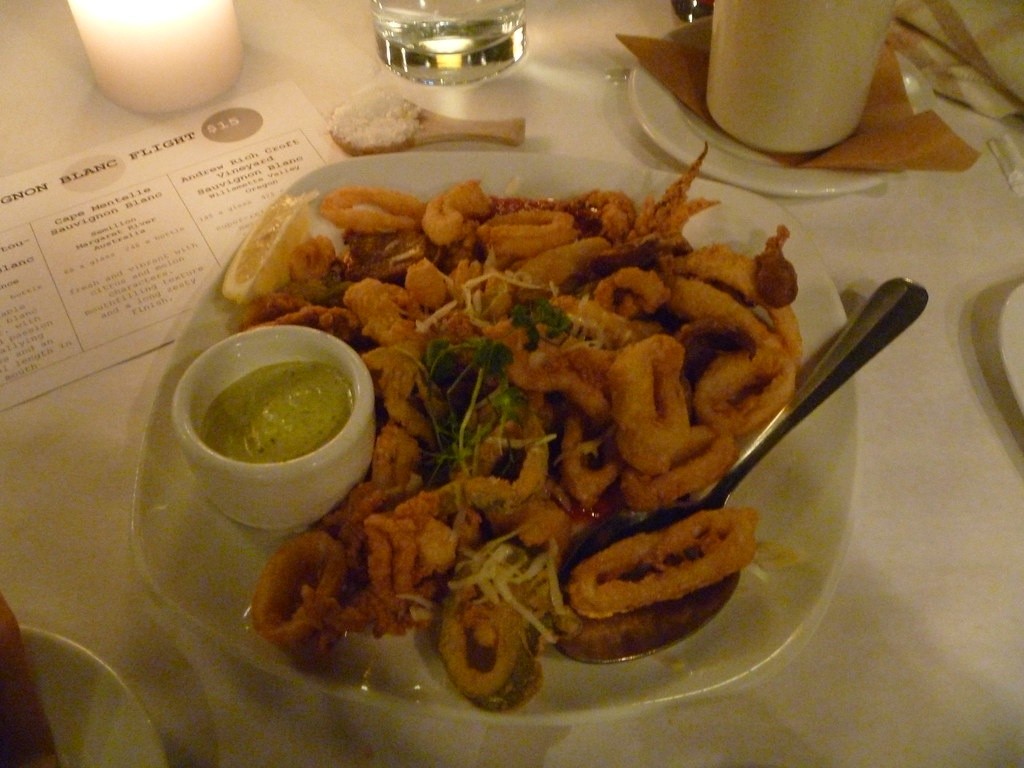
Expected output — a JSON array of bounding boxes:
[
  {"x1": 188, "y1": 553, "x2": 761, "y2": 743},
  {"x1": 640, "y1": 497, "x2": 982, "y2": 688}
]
[
  {"x1": 627, "y1": 16, "x2": 937, "y2": 198},
  {"x1": 19, "y1": 624, "x2": 168, "y2": 768},
  {"x1": 997, "y1": 284, "x2": 1024, "y2": 417},
  {"x1": 129, "y1": 149, "x2": 859, "y2": 728}
]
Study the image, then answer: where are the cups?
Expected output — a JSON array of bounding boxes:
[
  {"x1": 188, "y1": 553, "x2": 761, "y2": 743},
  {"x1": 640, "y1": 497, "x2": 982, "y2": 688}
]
[
  {"x1": 369, "y1": 0, "x2": 527, "y2": 87},
  {"x1": 705, "y1": 0, "x2": 895, "y2": 153}
]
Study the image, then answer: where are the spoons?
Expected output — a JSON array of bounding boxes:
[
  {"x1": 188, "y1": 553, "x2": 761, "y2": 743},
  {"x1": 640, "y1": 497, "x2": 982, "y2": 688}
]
[
  {"x1": 553, "y1": 276, "x2": 928, "y2": 666},
  {"x1": 672, "y1": 0, "x2": 714, "y2": 22},
  {"x1": 330, "y1": 95, "x2": 526, "y2": 154}
]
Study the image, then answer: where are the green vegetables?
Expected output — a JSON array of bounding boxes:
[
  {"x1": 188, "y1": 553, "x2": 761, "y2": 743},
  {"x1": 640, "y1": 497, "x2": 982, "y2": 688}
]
[{"x1": 412, "y1": 290, "x2": 572, "y2": 491}]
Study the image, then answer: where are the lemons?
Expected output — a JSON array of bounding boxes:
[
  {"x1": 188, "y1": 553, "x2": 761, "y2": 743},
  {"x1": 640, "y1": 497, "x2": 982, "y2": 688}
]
[{"x1": 220, "y1": 189, "x2": 315, "y2": 306}]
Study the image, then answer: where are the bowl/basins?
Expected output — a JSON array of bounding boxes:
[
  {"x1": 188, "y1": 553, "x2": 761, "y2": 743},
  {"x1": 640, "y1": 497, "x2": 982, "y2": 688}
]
[{"x1": 170, "y1": 325, "x2": 377, "y2": 531}]
